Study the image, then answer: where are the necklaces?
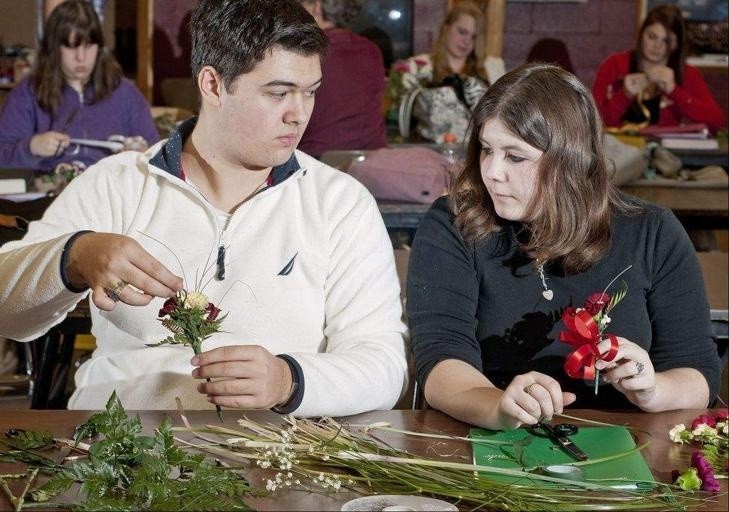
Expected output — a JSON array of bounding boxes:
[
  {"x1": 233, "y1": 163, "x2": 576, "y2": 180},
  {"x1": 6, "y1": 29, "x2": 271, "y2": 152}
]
[{"x1": 521, "y1": 223, "x2": 553, "y2": 301}]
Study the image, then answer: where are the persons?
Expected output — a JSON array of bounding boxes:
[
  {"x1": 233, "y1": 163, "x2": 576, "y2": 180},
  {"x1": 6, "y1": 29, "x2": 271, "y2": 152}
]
[
  {"x1": 0, "y1": 0, "x2": 409, "y2": 418},
  {"x1": 522, "y1": 38, "x2": 579, "y2": 77},
  {"x1": 590, "y1": 1, "x2": 729, "y2": 253},
  {"x1": 1, "y1": 0, "x2": 161, "y2": 171},
  {"x1": 405, "y1": 59, "x2": 724, "y2": 433},
  {"x1": 293, "y1": 0, "x2": 388, "y2": 159},
  {"x1": 384, "y1": 3, "x2": 491, "y2": 147}
]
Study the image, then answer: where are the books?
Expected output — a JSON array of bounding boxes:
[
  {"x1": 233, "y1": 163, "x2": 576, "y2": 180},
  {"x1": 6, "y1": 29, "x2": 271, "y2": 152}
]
[{"x1": 643, "y1": 120, "x2": 720, "y2": 151}]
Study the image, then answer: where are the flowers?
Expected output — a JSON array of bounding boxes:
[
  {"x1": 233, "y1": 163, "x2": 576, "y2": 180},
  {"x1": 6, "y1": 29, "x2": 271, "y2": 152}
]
[
  {"x1": 135, "y1": 228, "x2": 256, "y2": 423},
  {"x1": 561, "y1": 264, "x2": 634, "y2": 395},
  {"x1": 389, "y1": 57, "x2": 428, "y2": 96}
]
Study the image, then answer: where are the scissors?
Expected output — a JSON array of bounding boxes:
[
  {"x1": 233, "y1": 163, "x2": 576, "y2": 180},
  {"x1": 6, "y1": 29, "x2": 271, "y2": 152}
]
[{"x1": 526, "y1": 422, "x2": 589, "y2": 461}]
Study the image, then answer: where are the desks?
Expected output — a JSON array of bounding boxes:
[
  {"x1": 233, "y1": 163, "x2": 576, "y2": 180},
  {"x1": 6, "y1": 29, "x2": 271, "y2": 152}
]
[
  {"x1": 0, "y1": 165, "x2": 729, "y2": 228},
  {"x1": 0, "y1": 409, "x2": 728, "y2": 512},
  {"x1": 606, "y1": 126, "x2": 729, "y2": 153},
  {"x1": 669, "y1": 408, "x2": 727, "y2": 511}
]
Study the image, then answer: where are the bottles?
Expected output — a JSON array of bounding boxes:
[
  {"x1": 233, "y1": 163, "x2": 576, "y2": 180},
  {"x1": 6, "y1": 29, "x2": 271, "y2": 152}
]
[{"x1": 441, "y1": 134, "x2": 459, "y2": 160}]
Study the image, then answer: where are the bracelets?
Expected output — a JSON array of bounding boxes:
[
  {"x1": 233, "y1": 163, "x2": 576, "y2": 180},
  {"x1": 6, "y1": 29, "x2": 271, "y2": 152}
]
[{"x1": 275, "y1": 354, "x2": 298, "y2": 410}]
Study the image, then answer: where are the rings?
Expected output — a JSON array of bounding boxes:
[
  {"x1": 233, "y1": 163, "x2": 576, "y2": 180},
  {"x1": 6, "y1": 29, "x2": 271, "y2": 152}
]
[
  {"x1": 110, "y1": 278, "x2": 128, "y2": 296},
  {"x1": 634, "y1": 360, "x2": 645, "y2": 376},
  {"x1": 523, "y1": 381, "x2": 536, "y2": 394}
]
